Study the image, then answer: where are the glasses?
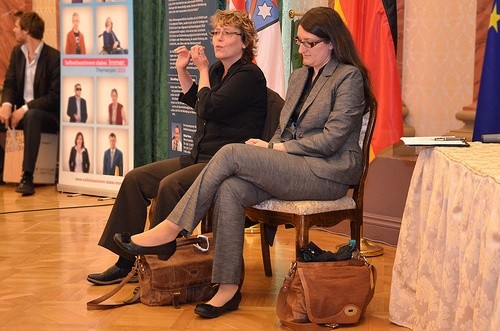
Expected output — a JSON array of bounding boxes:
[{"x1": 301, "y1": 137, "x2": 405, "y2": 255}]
[
  {"x1": 293, "y1": 36, "x2": 325, "y2": 48},
  {"x1": 76, "y1": 88, "x2": 81, "y2": 91},
  {"x1": 210, "y1": 28, "x2": 241, "y2": 38}
]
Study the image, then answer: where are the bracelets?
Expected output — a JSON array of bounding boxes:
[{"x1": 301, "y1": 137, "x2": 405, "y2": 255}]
[{"x1": 268, "y1": 141, "x2": 274, "y2": 150}]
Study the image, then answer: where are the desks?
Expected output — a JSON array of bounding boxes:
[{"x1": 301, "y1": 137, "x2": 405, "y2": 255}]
[{"x1": 389, "y1": 141, "x2": 500, "y2": 331}]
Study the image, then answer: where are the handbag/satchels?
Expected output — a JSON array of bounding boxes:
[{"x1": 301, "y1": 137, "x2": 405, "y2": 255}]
[
  {"x1": 87, "y1": 232, "x2": 245, "y2": 310},
  {"x1": 276, "y1": 240, "x2": 377, "y2": 331}
]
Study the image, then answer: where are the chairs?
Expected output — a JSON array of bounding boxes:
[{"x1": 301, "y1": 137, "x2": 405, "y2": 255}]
[{"x1": 149, "y1": 87, "x2": 393, "y2": 277}]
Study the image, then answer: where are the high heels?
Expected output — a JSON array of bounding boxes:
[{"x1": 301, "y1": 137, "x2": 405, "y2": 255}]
[
  {"x1": 194, "y1": 288, "x2": 242, "y2": 318},
  {"x1": 113, "y1": 233, "x2": 177, "y2": 261}
]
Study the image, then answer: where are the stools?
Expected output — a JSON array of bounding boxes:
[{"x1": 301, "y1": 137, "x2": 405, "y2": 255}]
[{"x1": 3, "y1": 129, "x2": 59, "y2": 184}]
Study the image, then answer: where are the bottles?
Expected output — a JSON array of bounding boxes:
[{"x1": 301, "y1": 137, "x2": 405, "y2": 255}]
[{"x1": 346, "y1": 240, "x2": 361, "y2": 261}]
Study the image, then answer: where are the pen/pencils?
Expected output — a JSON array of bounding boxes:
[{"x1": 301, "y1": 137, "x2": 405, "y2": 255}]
[{"x1": 435, "y1": 137, "x2": 466, "y2": 141}]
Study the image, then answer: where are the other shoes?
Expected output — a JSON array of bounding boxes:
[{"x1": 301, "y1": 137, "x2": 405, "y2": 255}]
[{"x1": 15, "y1": 169, "x2": 34, "y2": 194}]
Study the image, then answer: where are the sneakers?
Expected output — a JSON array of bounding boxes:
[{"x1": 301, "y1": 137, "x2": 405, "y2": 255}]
[{"x1": 86, "y1": 264, "x2": 139, "y2": 284}]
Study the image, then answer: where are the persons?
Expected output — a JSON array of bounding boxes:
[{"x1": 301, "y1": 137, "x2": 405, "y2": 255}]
[
  {"x1": 101, "y1": 132, "x2": 122, "y2": 176},
  {"x1": 103, "y1": 15, "x2": 129, "y2": 54},
  {"x1": 65, "y1": 12, "x2": 87, "y2": 55},
  {"x1": 0, "y1": 10, "x2": 61, "y2": 194},
  {"x1": 86, "y1": 13, "x2": 270, "y2": 290},
  {"x1": 66, "y1": 83, "x2": 88, "y2": 123},
  {"x1": 113, "y1": 6, "x2": 376, "y2": 320},
  {"x1": 107, "y1": 88, "x2": 128, "y2": 126},
  {"x1": 69, "y1": 131, "x2": 90, "y2": 174}
]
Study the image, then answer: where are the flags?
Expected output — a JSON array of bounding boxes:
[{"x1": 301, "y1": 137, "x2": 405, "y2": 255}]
[
  {"x1": 471, "y1": 0, "x2": 500, "y2": 146},
  {"x1": 328, "y1": 0, "x2": 407, "y2": 167},
  {"x1": 222, "y1": 0, "x2": 287, "y2": 102}
]
